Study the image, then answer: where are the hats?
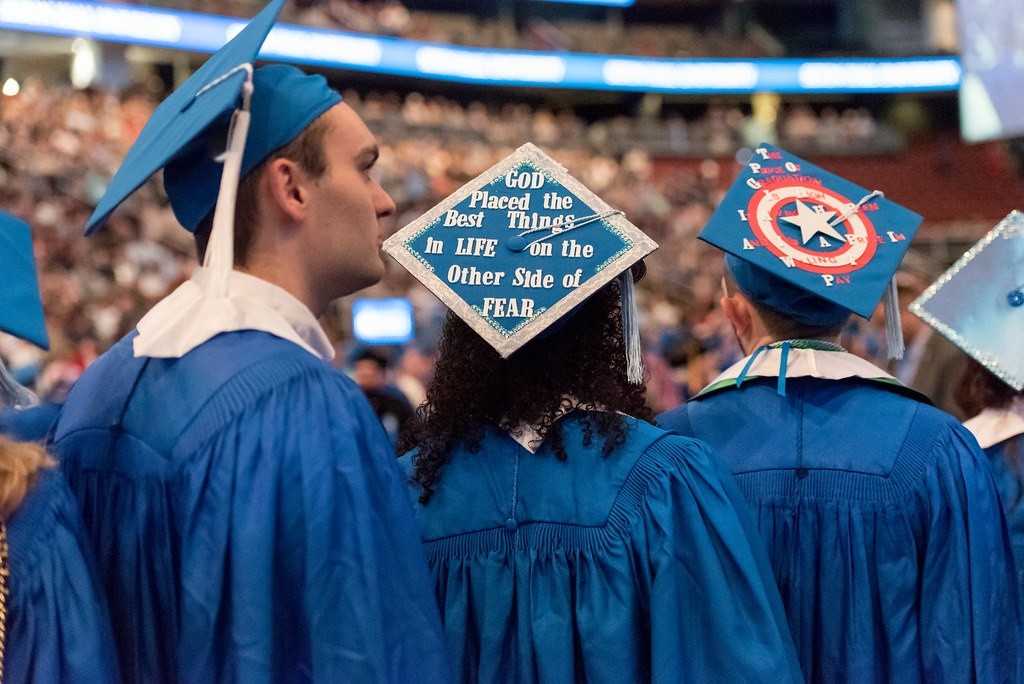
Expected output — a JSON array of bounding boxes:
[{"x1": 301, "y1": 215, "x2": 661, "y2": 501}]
[
  {"x1": 379, "y1": 143, "x2": 660, "y2": 385},
  {"x1": 0, "y1": 210, "x2": 49, "y2": 351},
  {"x1": 907, "y1": 209, "x2": 1024, "y2": 392},
  {"x1": 697, "y1": 142, "x2": 924, "y2": 361},
  {"x1": 83, "y1": 0, "x2": 343, "y2": 351}
]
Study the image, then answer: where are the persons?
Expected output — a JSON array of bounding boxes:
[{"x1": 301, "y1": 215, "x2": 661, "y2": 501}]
[
  {"x1": 380, "y1": 142, "x2": 806, "y2": 684},
  {"x1": 0, "y1": 0, "x2": 1024, "y2": 684},
  {"x1": 653, "y1": 142, "x2": 1016, "y2": 684},
  {"x1": 42, "y1": 0, "x2": 452, "y2": 684}
]
